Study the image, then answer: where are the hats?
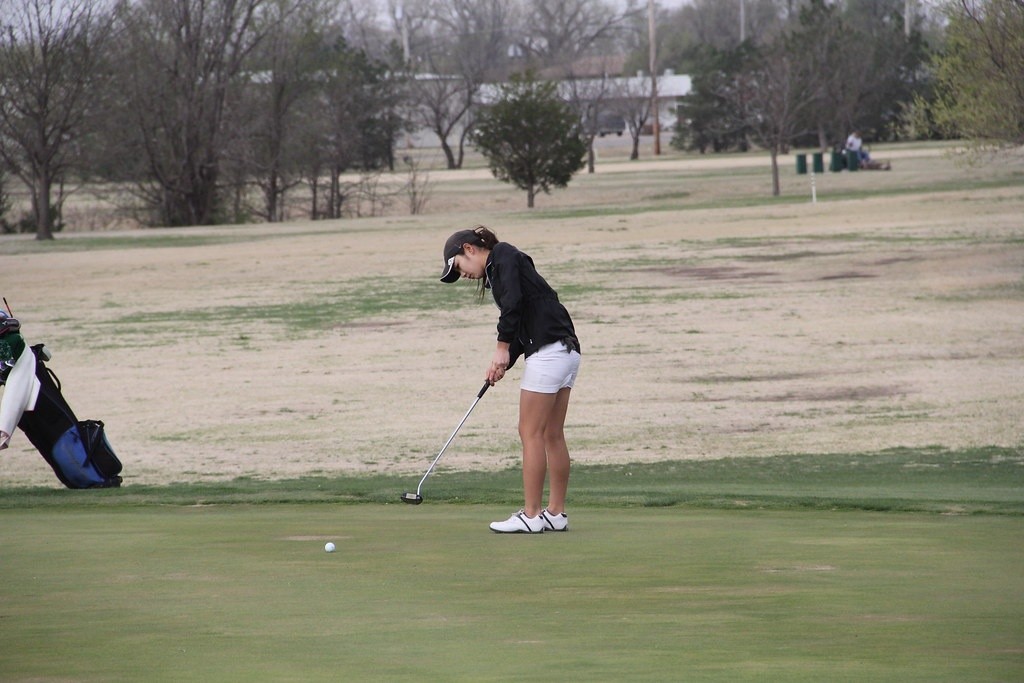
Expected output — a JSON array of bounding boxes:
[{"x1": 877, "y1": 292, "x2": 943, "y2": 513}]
[{"x1": 441, "y1": 229, "x2": 480, "y2": 284}]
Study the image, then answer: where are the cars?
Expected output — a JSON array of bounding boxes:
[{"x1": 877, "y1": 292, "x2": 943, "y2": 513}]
[{"x1": 638, "y1": 115, "x2": 664, "y2": 135}]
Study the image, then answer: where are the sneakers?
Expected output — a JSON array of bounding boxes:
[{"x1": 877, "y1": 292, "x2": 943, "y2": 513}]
[
  {"x1": 542, "y1": 506, "x2": 569, "y2": 532},
  {"x1": 489, "y1": 507, "x2": 545, "y2": 534}
]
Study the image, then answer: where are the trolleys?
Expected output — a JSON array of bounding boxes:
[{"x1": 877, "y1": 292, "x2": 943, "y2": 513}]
[{"x1": 0, "y1": 295, "x2": 123, "y2": 490}]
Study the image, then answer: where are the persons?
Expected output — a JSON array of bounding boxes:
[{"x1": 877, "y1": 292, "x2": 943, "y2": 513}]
[
  {"x1": 846, "y1": 130, "x2": 862, "y2": 170},
  {"x1": 439, "y1": 225, "x2": 581, "y2": 534}
]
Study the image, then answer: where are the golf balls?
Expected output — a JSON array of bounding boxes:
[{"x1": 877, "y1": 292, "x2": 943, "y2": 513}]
[{"x1": 324, "y1": 542, "x2": 335, "y2": 552}]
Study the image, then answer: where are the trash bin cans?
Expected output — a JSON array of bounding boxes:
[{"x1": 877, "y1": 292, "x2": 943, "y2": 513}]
[
  {"x1": 796, "y1": 153, "x2": 806, "y2": 173},
  {"x1": 813, "y1": 153, "x2": 823, "y2": 171}
]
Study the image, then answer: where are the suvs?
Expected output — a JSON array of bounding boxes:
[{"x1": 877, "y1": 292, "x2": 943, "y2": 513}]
[{"x1": 582, "y1": 110, "x2": 626, "y2": 137}]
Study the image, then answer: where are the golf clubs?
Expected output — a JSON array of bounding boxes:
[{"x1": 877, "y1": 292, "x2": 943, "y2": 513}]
[
  {"x1": 0, "y1": 358, "x2": 15, "y2": 381},
  {"x1": 400, "y1": 378, "x2": 490, "y2": 505}
]
